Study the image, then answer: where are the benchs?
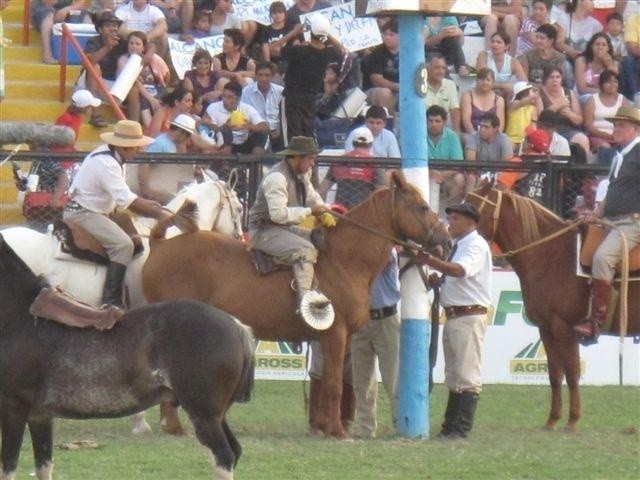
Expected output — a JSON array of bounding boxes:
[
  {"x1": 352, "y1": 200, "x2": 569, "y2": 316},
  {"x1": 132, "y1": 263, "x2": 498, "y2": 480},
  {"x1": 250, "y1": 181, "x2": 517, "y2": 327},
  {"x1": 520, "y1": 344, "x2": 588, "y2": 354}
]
[{"x1": 445, "y1": 31, "x2": 490, "y2": 100}]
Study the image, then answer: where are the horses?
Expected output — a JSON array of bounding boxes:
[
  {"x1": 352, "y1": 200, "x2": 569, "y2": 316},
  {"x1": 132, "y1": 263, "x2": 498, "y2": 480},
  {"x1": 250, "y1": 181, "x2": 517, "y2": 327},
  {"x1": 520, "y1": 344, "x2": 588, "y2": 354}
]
[
  {"x1": 0, "y1": 166, "x2": 247, "y2": 311},
  {"x1": 460, "y1": 176, "x2": 640, "y2": 432},
  {"x1": 0, "y1": 232, "x2": 257, "y2": 480},
  {"x1": 140, "y1": 169, "x2": 453, "y2": 444}
]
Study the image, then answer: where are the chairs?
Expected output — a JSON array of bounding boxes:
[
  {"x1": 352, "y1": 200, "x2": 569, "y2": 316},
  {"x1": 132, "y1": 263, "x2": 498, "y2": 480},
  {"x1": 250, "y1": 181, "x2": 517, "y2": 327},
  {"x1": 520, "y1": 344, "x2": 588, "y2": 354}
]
[{"x1": 52, "y1": 22, "x2": 100, "y2": 65}]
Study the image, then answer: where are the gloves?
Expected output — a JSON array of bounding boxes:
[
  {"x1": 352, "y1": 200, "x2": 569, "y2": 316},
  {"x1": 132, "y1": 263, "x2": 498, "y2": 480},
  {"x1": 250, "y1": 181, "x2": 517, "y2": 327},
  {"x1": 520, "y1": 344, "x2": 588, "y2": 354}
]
[{"x1": 319, "y1": 211, "x2": 337, "y2": 228}]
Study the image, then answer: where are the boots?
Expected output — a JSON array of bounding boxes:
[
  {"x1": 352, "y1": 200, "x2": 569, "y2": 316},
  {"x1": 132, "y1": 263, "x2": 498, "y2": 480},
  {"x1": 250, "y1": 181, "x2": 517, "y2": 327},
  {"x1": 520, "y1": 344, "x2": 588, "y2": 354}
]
[
  {"x1": 29, "y1": 287, "x2": 123, "y2": 333},
  {"x1": 451, "y1": 391, "x2": 479, "y2": 438},
  {"x1": 434, "y1": 392, "x2": 460, "y2": 438},
  {"x1": 292, "y1": 262, "x2": 331, "y2": 316},
  {"x1": 573, "y1": 278, "x2": 612, "y2": 340},
  {"x1": 56, "y1": 284, "x2": 111, "y2": 312}
]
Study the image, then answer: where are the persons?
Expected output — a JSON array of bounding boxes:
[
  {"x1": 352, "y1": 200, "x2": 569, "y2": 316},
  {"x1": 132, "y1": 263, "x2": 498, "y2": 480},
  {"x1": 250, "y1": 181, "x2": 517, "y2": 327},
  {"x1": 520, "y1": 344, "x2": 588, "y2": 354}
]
[
  {"x1": 417, "y1": 202, "x2": 492, "y2": 440},
  {"x1": 575, "y1": 108, "x2": 639, "y2": 344},
  {"x1": 65, "y1": 120, "x2": 164, "y2": 308},
  {"x1": 309, "y1": 246, "x2": 399, "y2": 440},
  {"x1": 19, "y1": 1, "x2": 639, "y2": 239},
  {"x1": 248, "y1": 136, "x2": 347, "y2": 315}
]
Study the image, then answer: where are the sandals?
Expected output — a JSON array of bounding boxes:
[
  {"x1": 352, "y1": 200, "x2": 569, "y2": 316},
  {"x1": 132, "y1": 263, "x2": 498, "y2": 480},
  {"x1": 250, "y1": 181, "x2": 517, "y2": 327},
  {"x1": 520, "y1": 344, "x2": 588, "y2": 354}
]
[{"x1": 88, "y1": 113, "x2": 106, "y2": 127}]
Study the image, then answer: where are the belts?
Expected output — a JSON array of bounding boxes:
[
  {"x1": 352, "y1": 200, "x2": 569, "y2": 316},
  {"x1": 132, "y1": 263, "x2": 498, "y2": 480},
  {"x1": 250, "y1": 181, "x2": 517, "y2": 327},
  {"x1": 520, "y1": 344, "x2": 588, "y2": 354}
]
[
  {"x1": 443, "y1": 305, "x2": 485, "y2": 319},
  {"x1": 370, "y1": 306, "x2": 396, "y2": 320}
]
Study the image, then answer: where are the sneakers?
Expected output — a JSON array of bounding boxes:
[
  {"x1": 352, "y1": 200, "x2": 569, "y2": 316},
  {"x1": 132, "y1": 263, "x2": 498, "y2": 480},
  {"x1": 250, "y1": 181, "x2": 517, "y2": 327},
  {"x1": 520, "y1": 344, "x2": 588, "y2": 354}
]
[{"x1": 455, "y1": 65, "x2": 470, "y2": 78}]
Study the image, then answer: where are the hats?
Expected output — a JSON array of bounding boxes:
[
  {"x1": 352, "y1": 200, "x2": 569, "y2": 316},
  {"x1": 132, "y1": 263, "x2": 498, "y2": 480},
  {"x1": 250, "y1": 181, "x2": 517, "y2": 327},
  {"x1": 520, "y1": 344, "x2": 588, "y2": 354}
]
[
  {"x1": 510, "y1": 81, "x2": 532, "y2": 103},
  {"x1": 445, "y1": 202, "x2": 478, "y2": 221},
  {"x1": 352, "y1": 126, "x2": 374, "y2": 144},
  {"x1": 525, "y1": 126, "x2": 549, "y2": 149},
  {"x1": 312, "y1": 12, "x2": 330, "y2": 37},
  {"x1": 99, "y1": 120, "x2": 154, "y2": 147},
  {"x1": 169, "y1": 114, "x2": 196, "y2": 135},
  {"x1": 70, "y1": 89, "x2": 102, "y2": 107},
  {"x1": 273, "y1": 136, "x2": 322, "y2": 155},
  {"x1": 95, "y1": 9, "x2": 123, "y2": 32},
  {"x1": 531, "y1": 110, "x2": 556, "y2": 126},
  {"x1": 603, "y1": 106, "x2": 640, "y2": 123}
]
